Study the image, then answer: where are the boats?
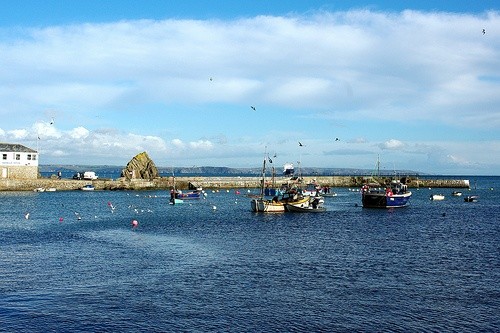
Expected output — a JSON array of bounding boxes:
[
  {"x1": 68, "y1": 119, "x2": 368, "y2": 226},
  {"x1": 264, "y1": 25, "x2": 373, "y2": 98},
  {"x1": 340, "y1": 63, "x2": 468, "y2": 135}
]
[
  {"x1": 80, "y1": 184, "x2": 95, "y2": 191},
  {"x1": 250, "y1": 157, "x2": 337, "y2": 213},
  {"x1": 168, "y1": 176, "x2": 206, "y2": 205},
  {"x1": 361, "y1": 153, "x2": 413, "y2": 208},
  {"x1": 451, "y1": 190, "x2": 463, "y2": 197},
  {"x1": 429, "y1": 194, "x2": 445, "y2": 201},
  {"x1": 33, "y1": 186, "x2": 57, "y2": 192},
  {"x1": 463, "y1": 195, "x2": 480, "y2": 202}
]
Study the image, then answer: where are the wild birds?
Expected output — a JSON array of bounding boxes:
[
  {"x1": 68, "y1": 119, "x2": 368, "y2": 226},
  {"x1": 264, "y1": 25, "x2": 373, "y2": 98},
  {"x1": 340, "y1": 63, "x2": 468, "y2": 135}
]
[
  {"x1": 297, "y1": 140, "x2": 303, "y2": 146},
  {"x1": 250, "y1": 105, "x2": 256, "y2": 111},
  {"x1": 482, "y1": 28, "x2": 486, "y2": 35},
  {"x1": 335, "y1": 137, "x2": 342, "y2": 142}
]
